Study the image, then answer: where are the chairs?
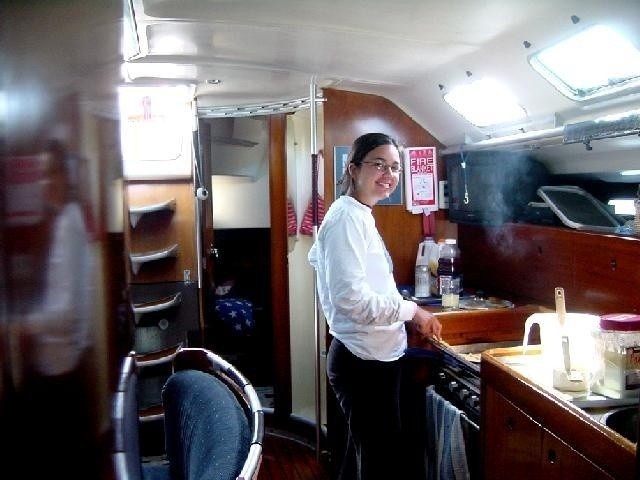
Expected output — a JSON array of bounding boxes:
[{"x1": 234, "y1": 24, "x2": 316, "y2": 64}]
[{"x1": 141, "y1": 345, "x2": 268, "y2": 480}]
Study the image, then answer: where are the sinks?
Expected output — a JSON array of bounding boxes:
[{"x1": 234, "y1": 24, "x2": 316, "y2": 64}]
[{"x1": 604, "y1": 405, "x2": 640, "y2": 445}]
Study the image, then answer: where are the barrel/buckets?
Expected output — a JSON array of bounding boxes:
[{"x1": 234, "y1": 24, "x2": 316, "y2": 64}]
[
  {"x1": 415, "y1": 236, "x2": 439, "y2": 266},
  {"x1": 430, "y1": 239, "x2": 444, "y2": 298}
]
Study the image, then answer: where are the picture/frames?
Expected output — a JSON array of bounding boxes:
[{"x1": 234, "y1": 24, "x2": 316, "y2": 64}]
[{"x1": 331, "y1": 143, "x2": 404, "y2": 207}]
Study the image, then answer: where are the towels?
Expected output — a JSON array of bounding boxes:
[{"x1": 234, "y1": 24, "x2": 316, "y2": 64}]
[{"x1": 425, "y1": 388, "x2": 471, "y2": 479}]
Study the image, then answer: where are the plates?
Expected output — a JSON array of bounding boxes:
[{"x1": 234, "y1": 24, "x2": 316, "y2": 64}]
[{"x1": 459, "y1": 298, "x2": 516, "y2": 312}]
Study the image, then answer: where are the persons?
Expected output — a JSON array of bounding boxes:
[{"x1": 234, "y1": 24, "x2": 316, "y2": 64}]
[{"x1": 307, "y1": 130, "x2": 443, "y2": 480}]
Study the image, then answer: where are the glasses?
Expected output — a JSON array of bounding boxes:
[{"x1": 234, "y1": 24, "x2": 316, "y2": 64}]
[{"x1": 359, "y1": 160, "x2": 402, "y2": 173}]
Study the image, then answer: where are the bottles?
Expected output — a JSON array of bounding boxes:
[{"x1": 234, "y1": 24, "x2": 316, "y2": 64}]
[
  {"x1": 413, "y1": 235, "x2": 463, "y2": 297},
  {"x1": 599, "y1": 313, "x2": 640, "y2": 400}
]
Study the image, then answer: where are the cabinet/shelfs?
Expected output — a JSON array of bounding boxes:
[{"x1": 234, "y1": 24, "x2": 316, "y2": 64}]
[
  {"x1": 125, "y1": 194, "x2": 191, "y2": 427},
  {"x1": 455, "y1": 221, "x2": 640, "y2": 318},
  {"x1": 478, "y1": 382, "x2": 637, "y2": 479}
]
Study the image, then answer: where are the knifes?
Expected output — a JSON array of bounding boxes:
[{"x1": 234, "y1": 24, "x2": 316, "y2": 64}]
[{"x1": 553, "y1": 286, "x2": 572, "y2": 375}]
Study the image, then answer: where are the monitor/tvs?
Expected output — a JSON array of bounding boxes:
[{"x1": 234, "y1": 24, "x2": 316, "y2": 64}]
[{"x1": 445, "y1": 150, "x2": 550, "y2": 223}]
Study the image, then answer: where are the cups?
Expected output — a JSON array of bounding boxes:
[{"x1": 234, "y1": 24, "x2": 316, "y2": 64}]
[{"x1": 439, "y1": 278, "x2": 460, "y2": 310}]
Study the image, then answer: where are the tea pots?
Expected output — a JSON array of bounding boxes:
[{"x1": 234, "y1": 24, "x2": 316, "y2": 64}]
[{"x1": 522, "y1": 311, "x2": 600, "y2": 393}]
